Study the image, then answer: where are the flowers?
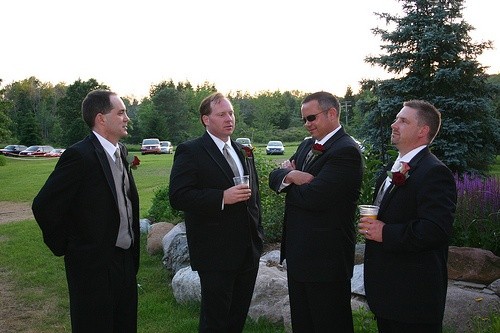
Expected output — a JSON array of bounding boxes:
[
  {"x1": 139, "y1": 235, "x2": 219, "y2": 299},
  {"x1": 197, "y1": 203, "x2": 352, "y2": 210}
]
[
  {"x1": 129, "y1": 155, "x2": 140, "y2": 174},
  {"x1": 241, "y1": 145, "x2": 255, "y2": 160},
  {"x1": 312, "y1": 143, "x2": 325, "y2": 157},
  {"x1": 387, "y1": 161, "x2": 410, "y2": 194}
]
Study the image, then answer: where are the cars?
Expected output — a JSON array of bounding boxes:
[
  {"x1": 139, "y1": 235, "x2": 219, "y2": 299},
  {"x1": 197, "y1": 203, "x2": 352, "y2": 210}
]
[
  {"x1": 44, "y1": 149, "x2": 66, "y2": 157},
  {"x1": 159, "y1": 141, "x2": 173, "y2": 154},
  {"x1": 140, "y1": 139, "x2": 161, "y2": 156},
  {"x1": 266, "y1": 141, "x2": 285, "y2": 155},
  {"x1": 0, "y1": 145, "x2": 28, "y2": 156},
  {"x1": 236, "y1": 138, "x2": 254, "y2": 152},
  {"x1": 19, "y1": 146, "x2": 54, "y2": 157}
]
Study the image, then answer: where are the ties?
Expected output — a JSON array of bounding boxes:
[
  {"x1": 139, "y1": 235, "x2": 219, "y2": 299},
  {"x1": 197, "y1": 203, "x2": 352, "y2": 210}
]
[
  {"x1": 301, "y1": 145, "x2": 315, "y2": 172},
  {"x1": 223, "y1": 144, "x2": 242, "y2": 186},
  {"x1": 114, "y1": 148, "x2": 123, "y2": 173},
  {"x1": 382, "y1": 161, "x2": 401, "y2": 192}
]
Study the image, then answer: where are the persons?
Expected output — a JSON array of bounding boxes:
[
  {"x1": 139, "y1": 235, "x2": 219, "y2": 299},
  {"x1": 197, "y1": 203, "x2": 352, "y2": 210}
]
[
  {"x1": 29, "y1": 89, "x2": 141, "y2": 333},
  {"x1": 268, "y1": 91, "x2": 364, "y2": 333},
  {"x1": 358, "y1": 97, "x2": 456, "y2": 333},
  {"x1": 168, "y1": 91, "x2": 265, "y2": 333}
]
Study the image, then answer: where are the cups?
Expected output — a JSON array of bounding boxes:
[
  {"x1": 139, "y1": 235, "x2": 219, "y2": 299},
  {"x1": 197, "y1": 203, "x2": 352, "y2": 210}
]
[
  {"x1": 359, "y1": 205, "x2": 379, "y2": 236},
  {"x1": 233, "y1": 175, "x2": 249, "y2": 189}
]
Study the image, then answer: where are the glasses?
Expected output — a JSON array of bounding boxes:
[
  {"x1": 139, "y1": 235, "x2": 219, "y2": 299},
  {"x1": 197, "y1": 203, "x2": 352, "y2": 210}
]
[{"x1": 302, "y1": 108, "x2": 330, "y2": 123}]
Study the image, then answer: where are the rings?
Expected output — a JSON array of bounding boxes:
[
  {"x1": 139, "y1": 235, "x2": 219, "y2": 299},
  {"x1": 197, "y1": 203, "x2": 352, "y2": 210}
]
[{"x1": 365, "y1": 229, "x2": 368, "y2": 234}]
